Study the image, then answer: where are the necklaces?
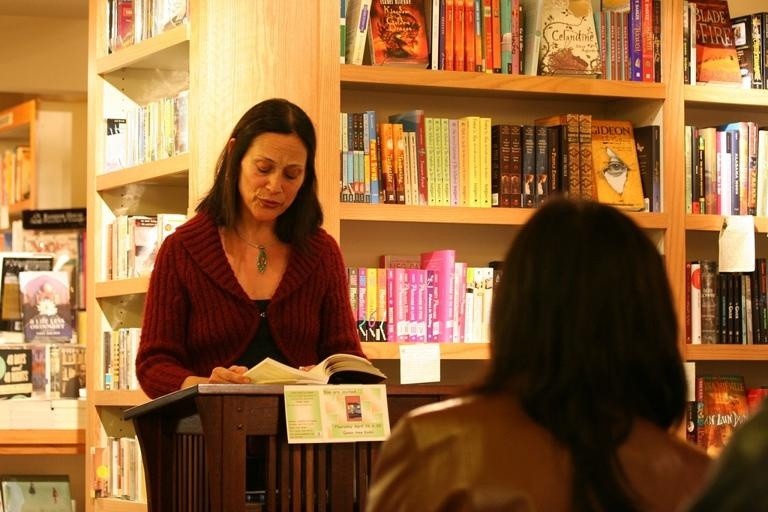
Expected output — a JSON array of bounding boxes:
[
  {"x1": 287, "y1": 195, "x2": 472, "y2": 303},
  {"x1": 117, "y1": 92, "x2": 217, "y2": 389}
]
[{"x1": 232, "y1": 224, "x2": 283, "y2": 274}]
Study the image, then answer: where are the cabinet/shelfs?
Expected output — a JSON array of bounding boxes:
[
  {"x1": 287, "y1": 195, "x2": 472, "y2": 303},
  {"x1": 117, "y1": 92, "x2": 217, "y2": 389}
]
[{"x1": 0, "y1": 2, "x2": 768, "y2": 512}]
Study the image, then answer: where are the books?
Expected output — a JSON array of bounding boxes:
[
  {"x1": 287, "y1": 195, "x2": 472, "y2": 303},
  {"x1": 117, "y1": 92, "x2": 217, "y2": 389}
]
[
  {"x1": 338, "y1": 110, "x2": 663, "y2": 213},
  {"x1": 685, "y1": 122, "x2": 768, "y2": 216},
  {"x1": 130, "y1": 90, "x2": 187, "y2": 164},
  {"x1": 341, "y1": 0, "x2": 660, "y2": 84},
  {"x1": 682, "y1": 0, "x2": 768, "y2": 89},
  {"x1": 107, "y1": 214, "x2": 187, "y2": 281},
  {"x1": 0, "y1": 146, "x2": 32, "y2": 207},
  {"x1": 0, "y1": 208, "x2": 87, "y2": 400},
  {"x1": 109, "y1": 0, "x2": 188, "y2": 54},
  {"x1": 345, "y1": 250, "x2": 504, "y2": 343},
  {"x1": 685, "y1": 258, "x2": 768, "y2": 345},
  {"x1": 683, "y1": 361, "x2": 768, "y2": 459},
  {"x1": 243, "y1": 352, "x2": 388, "y2": 390},
  {"x1": 103, "y1": 328, "x2": 142, "y2": 391}
]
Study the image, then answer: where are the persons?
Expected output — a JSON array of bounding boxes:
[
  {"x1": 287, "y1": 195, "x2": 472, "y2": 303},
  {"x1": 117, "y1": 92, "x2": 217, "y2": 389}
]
[
  {"x1": 357, "y1": 196, "x2": 730, "y2": 512},
  {"x1": 133, "y1": 97, "x2": 369, "y2": 404}
]
